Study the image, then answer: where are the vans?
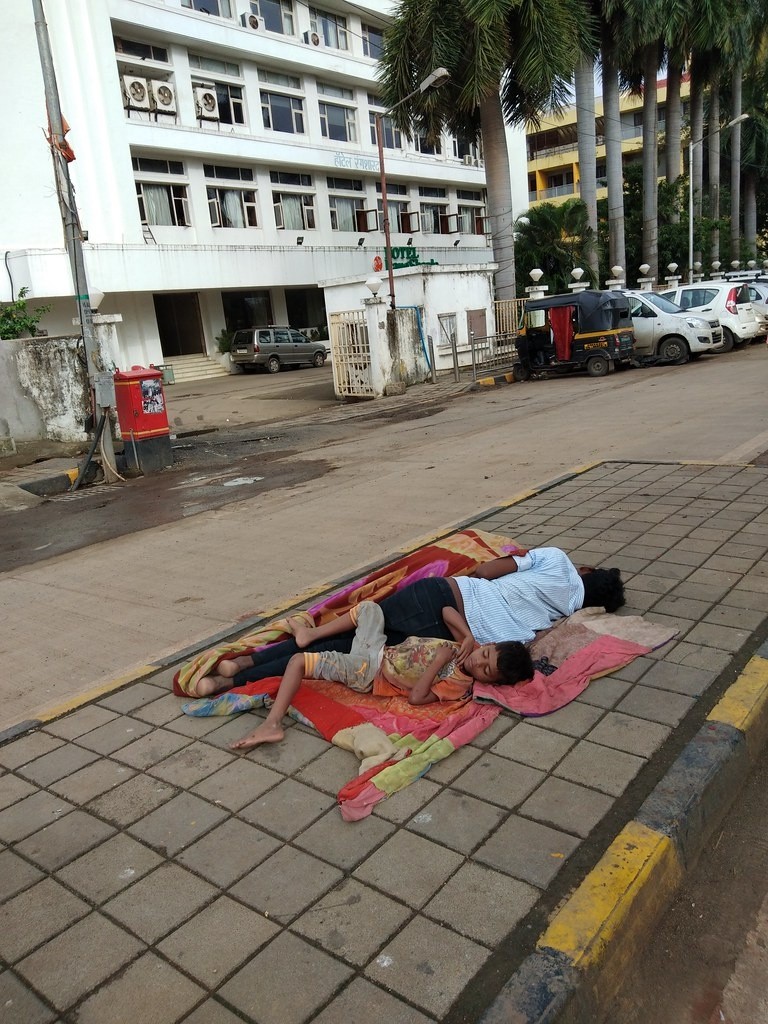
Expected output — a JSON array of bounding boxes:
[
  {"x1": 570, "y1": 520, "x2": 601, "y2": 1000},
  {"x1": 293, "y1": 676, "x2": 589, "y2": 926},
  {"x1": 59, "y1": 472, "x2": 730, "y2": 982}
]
[{"x1": 629, "y1": 282, "x2": 758, "y2": 354}]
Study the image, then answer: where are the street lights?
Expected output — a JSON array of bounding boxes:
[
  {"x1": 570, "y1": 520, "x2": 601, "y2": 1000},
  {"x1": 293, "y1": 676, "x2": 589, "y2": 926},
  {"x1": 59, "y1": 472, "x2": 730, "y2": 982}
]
[
  {"x1": 374, "y1": 66, "x2": 451, "y2": 310},
  {"x1": 689, "y1": 114, "x2": 751, "y2": 283}
]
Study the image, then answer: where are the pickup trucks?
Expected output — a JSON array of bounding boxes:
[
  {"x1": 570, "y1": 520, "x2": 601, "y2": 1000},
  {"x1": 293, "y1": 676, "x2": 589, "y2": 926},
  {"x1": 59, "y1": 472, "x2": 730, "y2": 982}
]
[{"x1": 230, "y1": 325, "x2": 327, "y2": 373}]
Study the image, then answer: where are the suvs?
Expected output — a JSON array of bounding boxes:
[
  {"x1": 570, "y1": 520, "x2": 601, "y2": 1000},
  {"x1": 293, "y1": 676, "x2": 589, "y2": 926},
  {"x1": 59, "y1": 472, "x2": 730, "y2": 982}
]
[{"x1": 613, "y1": 289, "x2": 723, "y2": 365}]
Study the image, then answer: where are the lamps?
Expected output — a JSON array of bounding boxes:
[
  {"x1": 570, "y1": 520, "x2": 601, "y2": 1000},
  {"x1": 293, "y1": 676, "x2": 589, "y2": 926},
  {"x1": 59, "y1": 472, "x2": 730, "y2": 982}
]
[
  {"x1": 572, "y1": 268, "x2": 583, "y2": 283},
  {"x1": 639, "y1": 264, "x2": 650, "y2": 277},
  {"x1": 407, "y1": 238, "x2": 414, "y2": 247},
  {"x1": 529, "y1": 268, "x2": 544, "y2": 285},
  {"x1": 731, "y1": 260, "x2": 740, "y2": 271},
  {"x1": 611, "y1": 266, "x2": 624, "y2": 280},
  {"x1": 296, "y1": 237, "x2": 303, "y2": 245},
  {"x1": 667, "y1": 263, "x2": 678, "y2": 276},
  {"x1": 693, "y1": 262, "x2": 702, "y2": 273},
  {"x1": 746, "y1": 260, "x2": 756, "y2": 270},
  {"x1": 711, "y1": 261, "x2": 721, "y2": 272},
  {"x1": 455, "y1": 240, "x2": 460, "y2": 247},
  {"x1": 88, "y1": 286, "x2": 105, "y2": 313},
  {"x1": 358, "y1": 238, "x2": 365, "y2": 246},
  {"x1": 365, "y1": 276, "x2": 382, "y2": 296},
  {"x1": 763, "y1": 260, "x2": 768, "y2": 268}
]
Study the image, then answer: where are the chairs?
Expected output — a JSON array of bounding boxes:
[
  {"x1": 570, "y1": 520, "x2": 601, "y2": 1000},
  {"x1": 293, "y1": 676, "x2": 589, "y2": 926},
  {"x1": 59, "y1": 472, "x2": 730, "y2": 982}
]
[
  {"x1": 640, "y1": 306, "x2": 651, "y2": 318},
  {"x1": 683, "y1": 297, "x2": 690, "y2": 308}
]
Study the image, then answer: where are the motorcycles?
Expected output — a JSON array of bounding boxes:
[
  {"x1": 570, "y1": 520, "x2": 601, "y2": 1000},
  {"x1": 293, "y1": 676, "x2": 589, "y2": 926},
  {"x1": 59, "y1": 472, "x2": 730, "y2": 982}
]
[{"x1": 511, "y1": 290, "x2": 636, "y2": 380}]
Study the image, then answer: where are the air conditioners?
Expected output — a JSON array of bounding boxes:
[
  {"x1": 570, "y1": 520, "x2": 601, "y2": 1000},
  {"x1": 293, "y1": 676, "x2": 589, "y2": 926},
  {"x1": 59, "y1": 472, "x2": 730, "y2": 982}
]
[
  {"x1": 303, "y1": 30, "x2": 325, "y2": 50},
  {"x1": 240, "y1": 12, "x2": 265, "y2": 30},
  {"x1": 192, "y1": 86, "x2": 219, "y2": 122},
  {"x1": 120, "y1": 75, "x2": 150, "y2": 112},
  {"x1": 146, "y1": 79, "x2": 177, "y2": 116},
  {"x1": 463, "y1": 155, "x2": 474, "y2": 166}
]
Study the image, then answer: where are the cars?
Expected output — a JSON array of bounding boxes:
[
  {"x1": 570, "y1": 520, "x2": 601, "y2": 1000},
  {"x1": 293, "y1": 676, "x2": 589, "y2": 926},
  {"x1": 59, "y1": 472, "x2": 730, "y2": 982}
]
[{"x1": 745, "y1": 284, "x2": 768, "y2": 328}]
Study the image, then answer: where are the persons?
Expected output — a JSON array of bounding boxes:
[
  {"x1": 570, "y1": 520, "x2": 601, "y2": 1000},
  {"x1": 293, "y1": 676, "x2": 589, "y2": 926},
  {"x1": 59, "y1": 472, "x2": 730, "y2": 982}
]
[{"x1": 194, "y1": 547, "x2": 628, "y2": 749}]
